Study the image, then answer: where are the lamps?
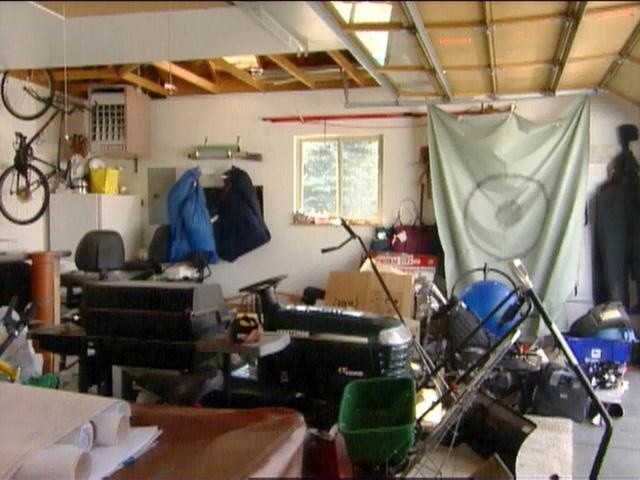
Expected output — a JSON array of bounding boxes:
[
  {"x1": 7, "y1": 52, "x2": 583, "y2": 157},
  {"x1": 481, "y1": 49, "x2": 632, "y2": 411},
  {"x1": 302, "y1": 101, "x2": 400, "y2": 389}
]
[{"x1": 458, "y1": 258, "x2": 613, "y2": 480}]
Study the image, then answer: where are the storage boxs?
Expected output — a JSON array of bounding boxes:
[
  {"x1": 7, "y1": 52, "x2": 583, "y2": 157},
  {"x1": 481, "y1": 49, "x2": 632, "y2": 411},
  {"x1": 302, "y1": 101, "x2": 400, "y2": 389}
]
[
  {"x1": 325, "y1": 259, "x2": 414, "y2": 319},
  {"x1": 554, "y1": 332, "x2": 630, "y2": 367}
]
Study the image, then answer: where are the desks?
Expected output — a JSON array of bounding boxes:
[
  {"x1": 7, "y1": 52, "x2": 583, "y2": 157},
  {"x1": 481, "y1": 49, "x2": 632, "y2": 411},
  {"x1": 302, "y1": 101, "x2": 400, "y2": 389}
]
[{"x1": 99, "y1": 404, "x2": 307, "y2": 480}]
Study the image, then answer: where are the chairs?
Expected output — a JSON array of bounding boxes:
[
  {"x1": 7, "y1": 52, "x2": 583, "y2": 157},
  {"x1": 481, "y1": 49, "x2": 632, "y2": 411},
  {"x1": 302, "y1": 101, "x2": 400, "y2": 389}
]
[
  {"x1": 59, "y1": 230, "x2": 125, "y2": 371},
  {"x1": 144, "y1": 225, "x2": 171, "y2": 272}
]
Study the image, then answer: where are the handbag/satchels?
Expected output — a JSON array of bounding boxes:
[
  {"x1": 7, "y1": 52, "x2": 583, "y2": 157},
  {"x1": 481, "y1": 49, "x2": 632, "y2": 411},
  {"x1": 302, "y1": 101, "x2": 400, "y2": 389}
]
[{"x1": 390, "y1": 225, "x2": 433, "y2": 252}]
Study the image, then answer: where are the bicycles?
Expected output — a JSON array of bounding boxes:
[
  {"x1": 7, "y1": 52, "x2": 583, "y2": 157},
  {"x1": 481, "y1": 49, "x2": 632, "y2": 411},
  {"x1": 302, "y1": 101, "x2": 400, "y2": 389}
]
[{"x1": 0, "y1": 70, "x2": 99, "y2": 224}]
